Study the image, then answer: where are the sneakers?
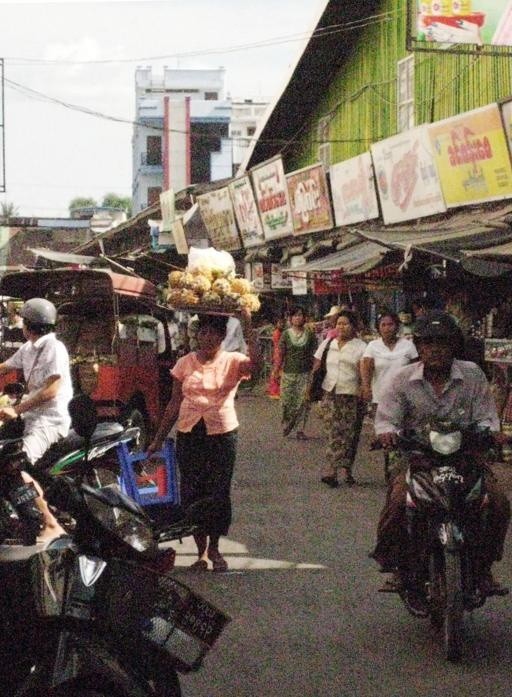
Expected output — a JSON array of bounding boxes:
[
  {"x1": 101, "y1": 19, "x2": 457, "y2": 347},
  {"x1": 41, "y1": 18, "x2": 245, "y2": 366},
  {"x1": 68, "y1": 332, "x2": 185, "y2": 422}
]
[
  {"x1": 283, "y1": 422, "x2": 306, "y2": 439},
  {"x1": 321, "y1": 474, "x2": 355, "y2": 485}
]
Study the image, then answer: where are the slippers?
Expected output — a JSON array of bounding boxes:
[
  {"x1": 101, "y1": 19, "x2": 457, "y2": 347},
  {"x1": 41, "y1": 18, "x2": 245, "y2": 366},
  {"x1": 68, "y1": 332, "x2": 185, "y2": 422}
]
[
  {"x1": 482, "y1": 572, "x2": 508, "y2": 595},
  {"x1": 378, "y1": 576, "x2": 406, "y2": 593},
  {"x1": 192, "y1": 553, "x2": 227, "y2": 572}
]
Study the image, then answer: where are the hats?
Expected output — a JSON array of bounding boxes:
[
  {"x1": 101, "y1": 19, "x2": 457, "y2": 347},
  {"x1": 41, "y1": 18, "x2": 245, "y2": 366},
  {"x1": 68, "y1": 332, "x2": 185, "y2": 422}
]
[
  {"x1": 413, "y1": 314, "x2": 458, "y2": 336},
  {"x1": 324, "y1": 305, "x2": 345, "y2": 317}
]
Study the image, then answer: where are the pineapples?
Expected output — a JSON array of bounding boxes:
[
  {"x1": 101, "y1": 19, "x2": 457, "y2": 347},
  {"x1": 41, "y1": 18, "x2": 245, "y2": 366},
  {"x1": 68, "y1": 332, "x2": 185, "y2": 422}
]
[{"x1": 161, "y1": 268, "x2": 262, "y2": 313}]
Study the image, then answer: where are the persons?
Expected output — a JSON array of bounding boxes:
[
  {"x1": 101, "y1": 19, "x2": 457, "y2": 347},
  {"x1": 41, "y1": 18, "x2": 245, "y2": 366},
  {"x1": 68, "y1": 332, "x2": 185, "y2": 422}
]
[
  {"x1": 354, "y1": 311, "x2": 418, "y2": 485},
  {"x1": 174, "y1": 303, "x2": 367, "y2": 443},
  {"x1": 373, "y1": 307, "x2": 511, "y2": 617},
  {"x1": 306, "y1": 309, "x2": 369, "y2": 486},
  {"x1": 143, "y1": 307, "x2": 265, "y2": 572},
  {"x1": 0, "y1": 297, "x2": 74, "y2": 551}
]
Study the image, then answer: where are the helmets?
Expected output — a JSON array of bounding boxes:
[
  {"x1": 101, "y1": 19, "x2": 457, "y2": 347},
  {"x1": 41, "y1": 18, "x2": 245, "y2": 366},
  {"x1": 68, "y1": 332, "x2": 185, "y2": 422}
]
[{"x1": 16, "y1": 298, "x2": 57, "y2": 325}]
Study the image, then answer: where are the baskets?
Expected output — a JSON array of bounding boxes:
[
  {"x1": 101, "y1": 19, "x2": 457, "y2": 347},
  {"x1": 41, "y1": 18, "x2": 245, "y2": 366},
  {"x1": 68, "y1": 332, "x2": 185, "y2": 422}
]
[{"x1": 108, "y1": 556, "x2": 232, "y2": 673}]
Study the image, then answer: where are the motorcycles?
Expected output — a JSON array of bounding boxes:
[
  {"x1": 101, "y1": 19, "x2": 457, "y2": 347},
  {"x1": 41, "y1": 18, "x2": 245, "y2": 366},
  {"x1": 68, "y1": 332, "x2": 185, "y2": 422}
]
[
  {"x1": 0, "y1": 267, "x2": 178, "y2": 457},
  {"x1": 369, "y1": 425, "x2": 512, "y2": 662},
  {"x1": 0, "y1": 383, "x2": 234, "y2": 697}
]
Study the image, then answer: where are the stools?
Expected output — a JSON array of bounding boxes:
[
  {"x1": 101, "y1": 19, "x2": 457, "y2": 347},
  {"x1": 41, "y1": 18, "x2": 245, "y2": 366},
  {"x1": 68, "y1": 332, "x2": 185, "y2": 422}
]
[{"x1": 116, "y1": 439, "x2": 183, "y2": 525}]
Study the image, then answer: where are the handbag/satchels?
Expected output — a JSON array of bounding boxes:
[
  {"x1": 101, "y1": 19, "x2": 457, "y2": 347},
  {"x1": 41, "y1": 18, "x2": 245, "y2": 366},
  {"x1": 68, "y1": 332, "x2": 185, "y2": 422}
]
[{"x1": 310, "y1": 368, "x2": 327, "y2": 401}]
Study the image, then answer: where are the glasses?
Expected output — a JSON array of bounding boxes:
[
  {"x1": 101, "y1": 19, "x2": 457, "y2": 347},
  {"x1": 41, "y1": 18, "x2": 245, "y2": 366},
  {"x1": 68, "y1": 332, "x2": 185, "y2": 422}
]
[{"x1": 417, "y1": 337, "x2": 452, "y2": 344}]
